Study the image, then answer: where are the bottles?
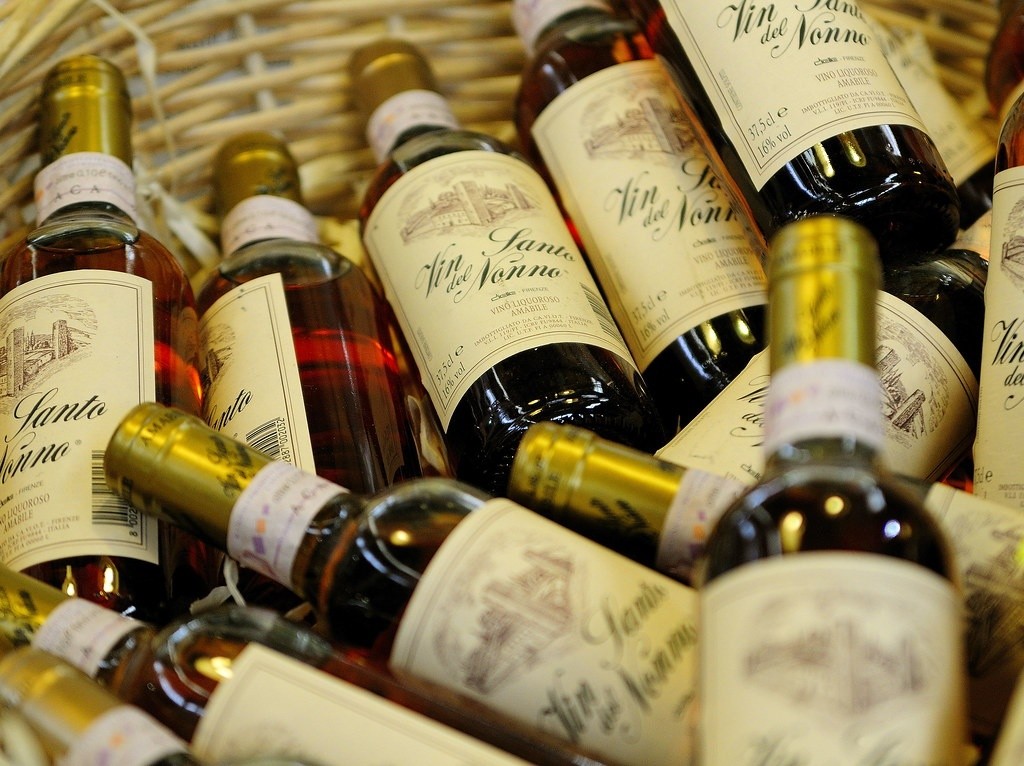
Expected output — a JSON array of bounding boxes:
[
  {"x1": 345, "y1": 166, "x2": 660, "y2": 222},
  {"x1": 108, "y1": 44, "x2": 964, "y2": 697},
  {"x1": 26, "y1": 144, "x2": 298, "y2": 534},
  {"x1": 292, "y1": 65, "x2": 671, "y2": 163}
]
[{"x1": 0, "y1": 0, "x2": 1024, "y2": 766}]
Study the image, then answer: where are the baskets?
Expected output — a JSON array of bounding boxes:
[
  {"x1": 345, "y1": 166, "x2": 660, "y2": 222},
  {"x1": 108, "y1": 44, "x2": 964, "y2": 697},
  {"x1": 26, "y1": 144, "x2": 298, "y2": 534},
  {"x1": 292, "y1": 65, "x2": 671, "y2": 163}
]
[{"x1": 0, "y1": 0, "x2": 1003, "y2": 281}]
